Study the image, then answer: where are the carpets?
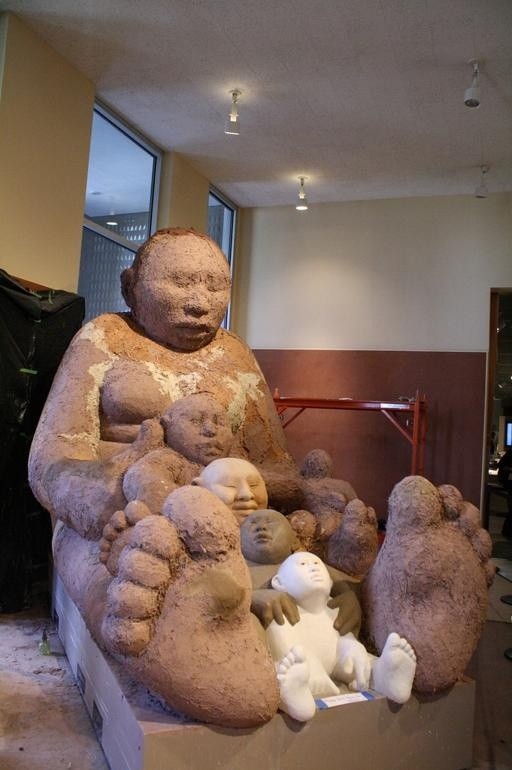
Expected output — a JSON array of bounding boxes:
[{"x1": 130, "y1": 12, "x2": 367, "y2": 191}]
[{"x1": 481, "y1": 556, "x2": 511, "y2": 624}]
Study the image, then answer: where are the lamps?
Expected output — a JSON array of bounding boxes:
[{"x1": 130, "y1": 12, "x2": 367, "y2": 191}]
[
  {"x1": 224, "y1": 84, "x2": 249, "y2": 140},
  {"x1": 292, "y1": 171, "x2": 315, "y2": 215},
  {"x1": 496, "y1": 321, "x2": 506, "y2": 335},
  {"x1": 467, "y1": 157, "x2": 498, "y2": 200},
  {"x1": 496, "y1": 374, "x2": 511, "y2": 390},
  {"x1": 461, "y1": 54, "x2": 486, "y2": 111}
]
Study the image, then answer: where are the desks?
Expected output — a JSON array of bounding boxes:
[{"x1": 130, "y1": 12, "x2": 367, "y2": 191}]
[{"x1": 265, "y1": 389, "x2": 433, "y2": 491}]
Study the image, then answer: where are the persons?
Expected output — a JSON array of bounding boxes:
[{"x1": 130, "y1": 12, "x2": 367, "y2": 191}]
[
  {"x1": 178, "y1": 456, "x2": 271, "y2": 527},
  {"x1": 235, "y1": 507, "x2": 368, "y2": 637},
  {"x1": 26, "y1": 225, "x2": 495, "y2": 728},
  {"x1": 259, "y1": 551, "x2": 417, "y2": 720},
  {"x1": 96, "y1": 391, "x2": 378, "y2": 583}
]
